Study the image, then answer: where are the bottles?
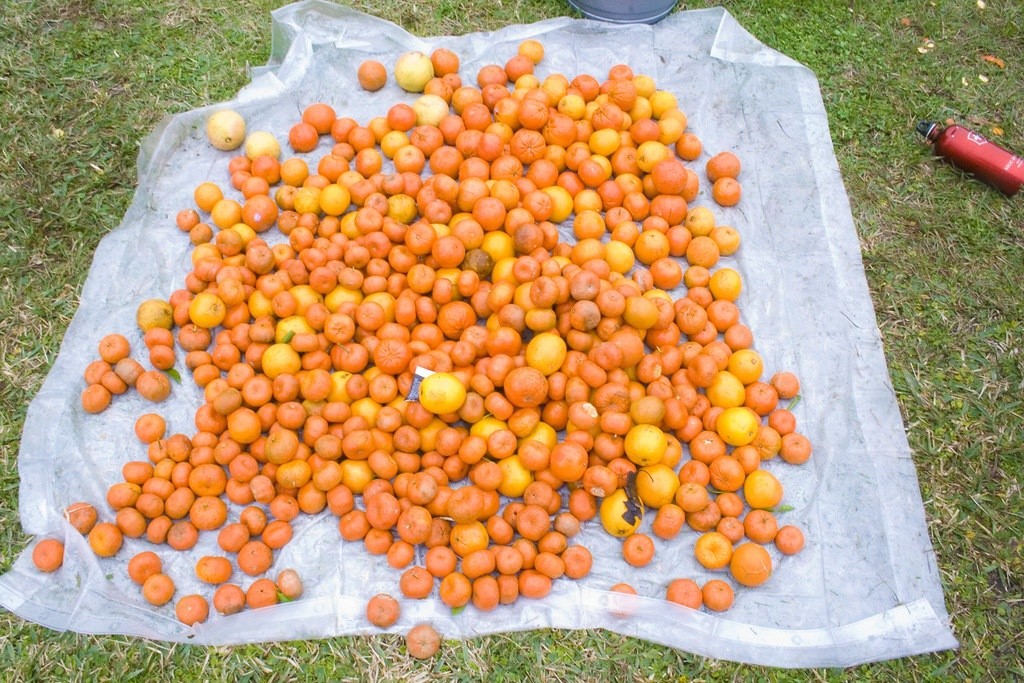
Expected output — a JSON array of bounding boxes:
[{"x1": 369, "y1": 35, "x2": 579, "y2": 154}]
[{"x1": 916, "y1": 119, "x2": 1024, "y2": 197}]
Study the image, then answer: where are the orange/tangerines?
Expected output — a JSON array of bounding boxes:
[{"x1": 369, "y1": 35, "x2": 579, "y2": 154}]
[{"x1": 33, "y1": 40, "x2": 812, "y2": 660}]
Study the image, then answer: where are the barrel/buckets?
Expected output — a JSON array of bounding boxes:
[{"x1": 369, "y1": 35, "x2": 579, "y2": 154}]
[{"x1": 566, "y1": 0, "x2": 677, "y2": 25}]
